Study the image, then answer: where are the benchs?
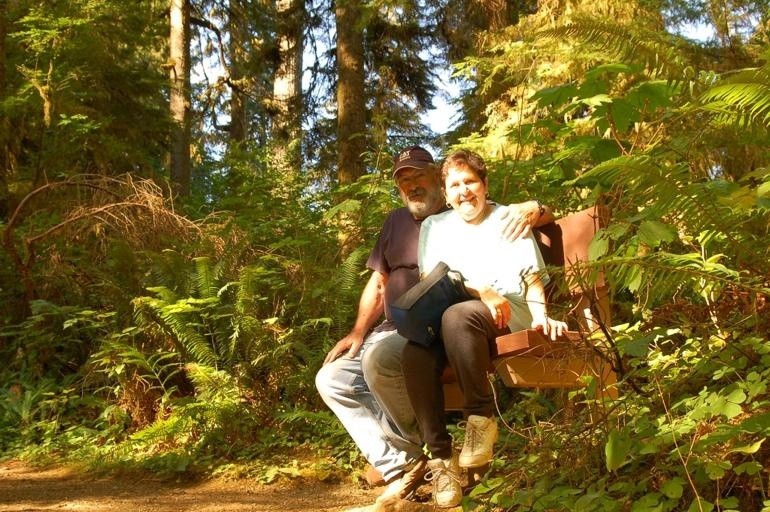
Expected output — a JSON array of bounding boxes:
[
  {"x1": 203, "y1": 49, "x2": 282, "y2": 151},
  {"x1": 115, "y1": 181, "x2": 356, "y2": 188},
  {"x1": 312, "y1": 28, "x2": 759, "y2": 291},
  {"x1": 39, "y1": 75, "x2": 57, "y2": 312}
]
[{"x1": 438, "y1": 203, "x2": 619, "y2": 412}]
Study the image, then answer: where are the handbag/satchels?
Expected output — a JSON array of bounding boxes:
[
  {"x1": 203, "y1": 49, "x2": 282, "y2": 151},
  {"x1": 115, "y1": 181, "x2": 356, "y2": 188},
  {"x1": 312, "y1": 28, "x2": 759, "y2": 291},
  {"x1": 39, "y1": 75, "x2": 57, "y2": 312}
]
[{"x1": 388, "y1": 261, "x2": 474, "y2": 346}]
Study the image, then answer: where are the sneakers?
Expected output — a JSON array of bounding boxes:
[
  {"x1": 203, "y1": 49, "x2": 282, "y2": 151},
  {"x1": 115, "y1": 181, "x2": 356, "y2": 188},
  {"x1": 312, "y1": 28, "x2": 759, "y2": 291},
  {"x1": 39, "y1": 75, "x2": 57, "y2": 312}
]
[
  {"x1": 364, "y1": 452, "x2": 462, "y2": 507},
  {"x1": 458, "y1": 414, "x2": 499, "y2": 468}
]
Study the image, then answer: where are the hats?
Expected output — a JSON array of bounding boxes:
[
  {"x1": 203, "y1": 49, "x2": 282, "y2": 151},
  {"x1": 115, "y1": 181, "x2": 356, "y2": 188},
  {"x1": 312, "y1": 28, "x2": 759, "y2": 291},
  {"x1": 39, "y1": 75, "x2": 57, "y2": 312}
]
[{"x1": 392, "y1": 144, "x2": 435, "y2": 180}]
